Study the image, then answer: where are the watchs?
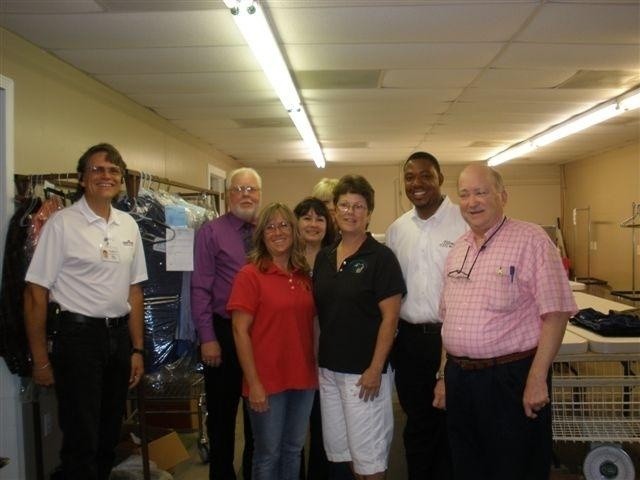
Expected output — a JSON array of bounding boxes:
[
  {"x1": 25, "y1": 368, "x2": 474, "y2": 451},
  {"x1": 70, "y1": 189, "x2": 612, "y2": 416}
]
[{"x1": 436, "y1": 370, "x2": 445, "y2": 380}]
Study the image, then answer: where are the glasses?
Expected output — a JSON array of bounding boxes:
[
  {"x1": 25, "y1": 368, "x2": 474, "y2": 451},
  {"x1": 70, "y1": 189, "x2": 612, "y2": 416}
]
[
  {"x1": 264, "y1": 222, "x2": 289, "y2": 233},
  {"x1": 447, "y1": 246, "x2": 483, "y2": 280},
  {"x1": 88, "y1": 166, "x2": 121, "y2": 175},
  {"x1": 336, "y1": 199, "x2": 367, "y2": 213},
  {"x1": 226, "y1": 184, "x2": 261, "y2": 192}
]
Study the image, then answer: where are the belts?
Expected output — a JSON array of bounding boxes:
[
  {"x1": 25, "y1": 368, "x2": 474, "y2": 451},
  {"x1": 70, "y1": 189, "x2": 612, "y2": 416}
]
[
  {"x1": 59, "y1": 310, "x2": 130, "y2": 327},
  {"x1": 446, "y1": 346, "x2": 538, "y2": 370},
  {"x1": 398, "y1": 318, "x2": 443, "y2": 334}
]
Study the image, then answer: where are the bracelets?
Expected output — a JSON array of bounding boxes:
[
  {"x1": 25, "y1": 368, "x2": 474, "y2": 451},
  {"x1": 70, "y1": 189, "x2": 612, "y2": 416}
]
[
  {"x1": 131, "y1": 347, "x2": 147, "y2": 357},
  {"x1": 32, "y1": 360, "x2": 51, "y2": 371}
]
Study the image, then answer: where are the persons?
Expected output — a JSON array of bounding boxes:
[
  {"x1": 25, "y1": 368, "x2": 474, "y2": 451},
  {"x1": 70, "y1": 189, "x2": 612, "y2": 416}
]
[
  {"x1": 189, "y1": 166, "x2": 265, "y2": 480},
  {"x1": 383, "y1": 151, "x2": 470, "y2": 480},
  {"x1": 432, "y1": 165, "x2": 579, "y2": 480},
  {"x1": 226, "y1": 201, "x2": 319, "y2": 480},
  {"x1": 292, "y1": 200, "x2": 336, "y2": 480},
  {"x1": 312, "y1": 175, "x2": 407, "y2": 480},
  {"x1": 24, "y1": 143, "x2": 149, "y2": 480},
  {"x1": 313, "y1": 179, "x2": 343, "y2": 246}
]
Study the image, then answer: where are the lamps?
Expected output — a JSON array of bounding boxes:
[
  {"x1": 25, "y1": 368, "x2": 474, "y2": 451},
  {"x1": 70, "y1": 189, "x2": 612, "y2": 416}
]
[
  {"x1": 221, "y1": 0, "x2": 326, "y2": 169},
  {"x1": 486, "y1": 85, "x2": 640, "y2": 167}
]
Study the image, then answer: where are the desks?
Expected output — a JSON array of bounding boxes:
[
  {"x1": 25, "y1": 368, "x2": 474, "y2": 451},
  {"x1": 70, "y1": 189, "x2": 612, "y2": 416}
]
[{"x1": 547, "y1": 281, "x2": 640, "y2": 419}]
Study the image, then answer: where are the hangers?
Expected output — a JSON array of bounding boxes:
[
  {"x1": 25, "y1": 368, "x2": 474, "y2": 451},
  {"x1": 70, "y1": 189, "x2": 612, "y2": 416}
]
[
  {"x1": 619, "y1": 204, "x2": 640, "y2": 228},
  {"x1": 17, "y1": 167, "x2": 177, "y2": 245}
]
